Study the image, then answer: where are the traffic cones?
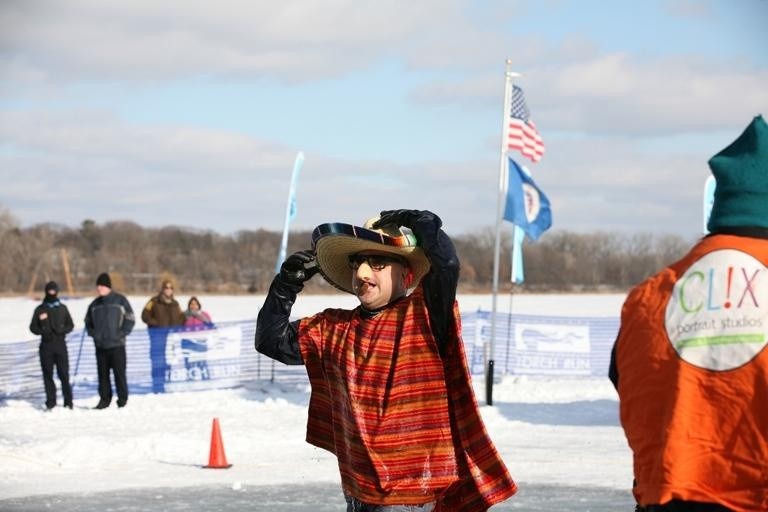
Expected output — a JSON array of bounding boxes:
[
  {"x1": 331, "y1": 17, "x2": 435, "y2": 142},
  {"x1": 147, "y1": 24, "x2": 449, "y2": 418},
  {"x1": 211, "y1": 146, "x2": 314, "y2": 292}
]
[{"x1": 203, "y1": 418, "x2": 233, "y2": 467}]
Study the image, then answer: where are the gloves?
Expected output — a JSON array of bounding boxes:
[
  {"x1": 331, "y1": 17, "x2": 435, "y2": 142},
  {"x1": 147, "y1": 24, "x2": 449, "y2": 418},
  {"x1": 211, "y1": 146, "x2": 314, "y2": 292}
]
[
  {"x1": 373, "y1": 210, "x2": 414, "y2": 229},
  {"x1": 280, "y1": 249, "x2": 318, "y2": 282}
]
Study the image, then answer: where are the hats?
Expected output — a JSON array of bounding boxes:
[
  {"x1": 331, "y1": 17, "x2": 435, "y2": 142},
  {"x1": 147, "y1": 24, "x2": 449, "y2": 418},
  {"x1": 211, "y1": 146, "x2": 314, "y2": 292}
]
[
  {"x1": 97, "y1": 273, "x2": 111, "y2": 288},
  {"x1": 45, "y1": 281, "x2": 59, "y2": 296},
  {"x1": 708, "y1": 114, "x2": 764, "y2": 229},
  {"x1": 311, "y1": 216, "x2": 430, "y2": 295}
]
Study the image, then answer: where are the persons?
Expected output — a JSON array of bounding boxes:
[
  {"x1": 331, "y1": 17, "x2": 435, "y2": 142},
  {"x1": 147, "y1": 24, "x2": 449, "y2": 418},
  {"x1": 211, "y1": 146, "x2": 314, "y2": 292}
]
[
  {"x1": 252, "y1": 209, "x2": 522, "y2": 512},
  {"x1": 29, "y1": 280, "x2": 75, "y2": 412},
  {"x1": 178, "y1": 296, "x2": 213, "y2": 382},
  {"x1": 83, "y1": 272, "x2": 136, "y2": 410},
  {"x1": 140, "y1": 280, "x2": 185, "y2": 394},
  {"x1": 608, "y1": 111, "x2": 768, "y2": 512}
]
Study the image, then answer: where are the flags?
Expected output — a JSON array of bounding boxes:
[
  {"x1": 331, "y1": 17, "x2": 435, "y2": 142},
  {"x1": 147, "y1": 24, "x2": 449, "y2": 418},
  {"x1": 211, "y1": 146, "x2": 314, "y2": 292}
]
[
  {"x1": 506, "y1": 85, "x2": 545, "y2": 163},
  {"x1": 500, "y1": 157, "x2": 555, "y2": 242}
]
